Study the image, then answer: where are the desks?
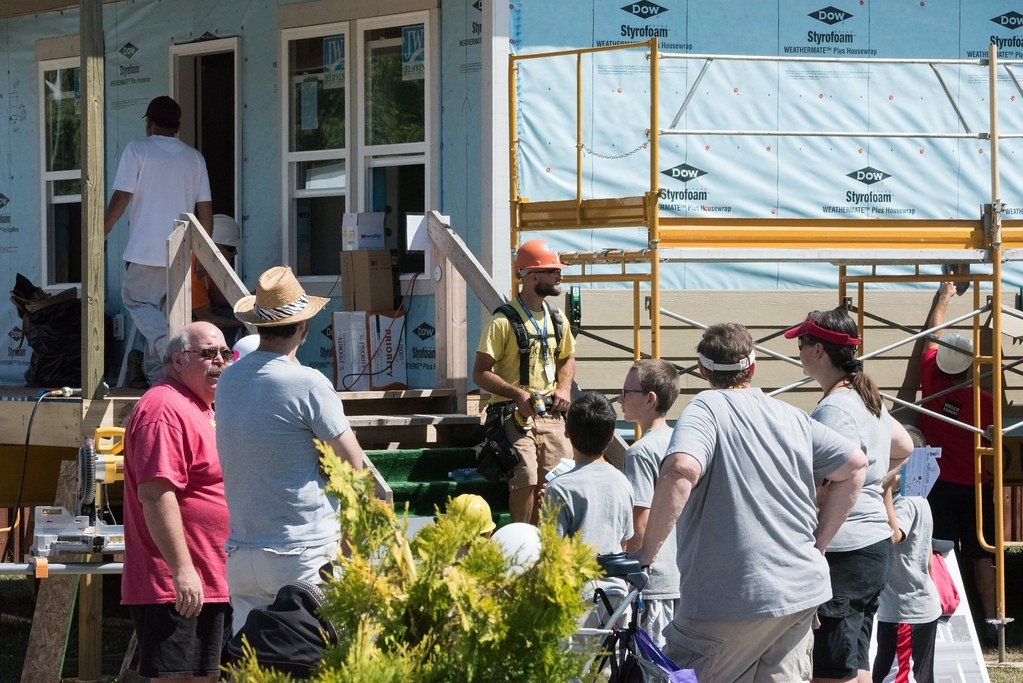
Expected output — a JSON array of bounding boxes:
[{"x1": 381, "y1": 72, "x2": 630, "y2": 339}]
[{"x1": 0, "y1": 556, "x2": 123, "y2": 682}]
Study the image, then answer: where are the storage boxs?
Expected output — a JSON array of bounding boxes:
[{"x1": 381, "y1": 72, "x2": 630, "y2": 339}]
[
  {"x1": 331, "y1": 311, "x2": 407, "y2": 391},
  {"x1": 341, "y1": 211, "x2": 386, "y2": 251},
  {"x1": 338, "y1": 251, "x2": 395, "y2": 312}
]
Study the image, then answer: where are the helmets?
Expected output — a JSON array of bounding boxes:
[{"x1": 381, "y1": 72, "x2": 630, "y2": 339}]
[
  {"x1": 512, "y1": 240, "x2": 567, "y2": 270},
  {"x1": 937, "y1": 334, "x2": 974, "y2": 374},
  {"x1": 451, "y1": 492, "x2": 496, "y2": 535}
]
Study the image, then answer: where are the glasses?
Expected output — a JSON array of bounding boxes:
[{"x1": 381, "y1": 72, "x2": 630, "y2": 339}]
[
  {"x1": 621, "y1": 388, "x2": 647, "y2": 398},
  {"x1": 182, "y1": 347, "x2": 234, "y2": 360},
  {"x1": 527, "y1": 269, "x2": 561, "y2": 275},
  {"x1": 797, "y1": 337, "x2": 818, "y2": 351}
]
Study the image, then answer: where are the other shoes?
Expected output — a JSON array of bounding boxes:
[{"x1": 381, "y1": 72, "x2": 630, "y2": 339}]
[{"x1": 979, "y1": 616, "x2": 1000, "y2": 647}]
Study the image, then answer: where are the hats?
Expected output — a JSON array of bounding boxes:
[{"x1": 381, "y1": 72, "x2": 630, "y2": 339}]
[
  {"x1": 784, "y1": 318, "x2": 862, "y2": 346},
  {"x1": 234, "y1": 266, "x2": 331, "y2": 326},
  {"x1": 141, "y1": 96, "x2": 181, "y2": 128}
]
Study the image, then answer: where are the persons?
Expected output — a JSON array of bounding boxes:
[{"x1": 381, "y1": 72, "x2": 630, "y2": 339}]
[
  {"x1": 106, "y1": 94, "x2": 212, "y2": 388},
  {"x1": 870, "y1": 422, "x2": 943, "y2": 683},
  {"x1": 440, "y1": 359, "x2": 681, "y2": 683},
  {"x1": 784, "y1": 310, "x2": 916, "y2": 683},
  {"x1": 119, "y1": 321, "x2": 230, "y2": 683},
  {"x1": 627, "y1": 322, "x2": 868, "y2": 683},
  {"x1": 215, "y1": 266, "x2": 364, "y2": 680},
  {"x1": 192, "y1": 214, "x2": 256, "y2": 345},
  {"x1": 919, "y1": 270, "x2": 1006, "y2": 646},
  {"x1": 471, "y1": 240, "x2": 577, "y2": 530}
]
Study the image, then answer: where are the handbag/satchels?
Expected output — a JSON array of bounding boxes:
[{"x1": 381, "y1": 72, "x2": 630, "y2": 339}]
[
  {"x1": 607, "y1": 628, "x2": 700, "y2": 683},
  {"x1": 474, "y1": 428, "x2": 522, "y2": 493}
]
